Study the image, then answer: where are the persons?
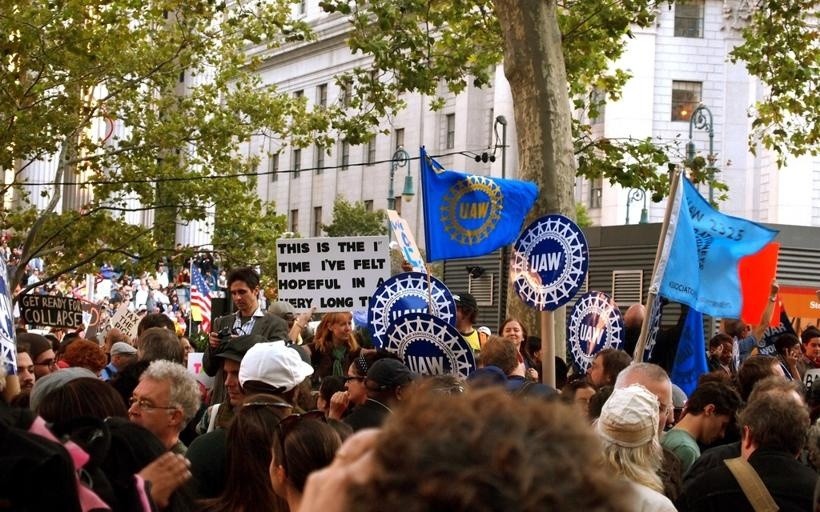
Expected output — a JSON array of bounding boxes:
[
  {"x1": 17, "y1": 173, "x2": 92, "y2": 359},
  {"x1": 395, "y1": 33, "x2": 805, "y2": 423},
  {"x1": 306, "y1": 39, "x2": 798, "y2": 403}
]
[
  {"x1": 499, "y1": 318, "x2": 541, "y2": 381},
  {"x1": 315, "y1": 377, "x2": 344, "y2": 412},
  {"x1": 328, "y1": 352, "x2": 381, "y2": 424},
  {"x1": 268, "y1": 415, "x2": 343, "y2": 512},
  {"x1": 559, "y1": 348, "x2": 632, "y2": 419},
  {"x1": 0, "y1": 239, "x2": 76, "y2": 299},
  {"x1": 526, "y1": 336, "x2": 541, "y2": 366},
  {"x1": 194, "y1": 334, "x2": 268, "y2": 434},
  {"x1": 452, "y1": 292, "x2": 487, "y2": 367},
  {"x1": 464, "y1": 365, "x2": 506, "y2": 392},
  {"x1": 204, "y1": 270, "x2": 216, "y2": 291},
  {"x1": 614, "y1": 362, "x2": 672, "y2": 439},
  {"x1": 217, "y1": 270, "x2": 228, "y2": 291},
  {"x1": 104, "y1": 259, "x2": 190, "y2": 336},
  {"x1": 661, "y1": 382, "x2": 740, "y2": 477},
  {"x1": 288, "y1": 306, "x2": 376, "y2": 383},
  {"x1": 201, "y1": 268, "x2": 287, "y2": 406},
  {"x1": 798, "y1": 328, "x2": 820, "y2": 376},
  {"x1": 707, "y1": 333, "x2": 736, "y2": 375},
  {"x1": 698, "y1": 354, "x2": 820, "y2": 403},
  {"x1": 344, "y1": 358, "x2": 416, "y2": 428},
  {"x1": 676, "y1": 387, "x2": 820, "y2": 512},
  {"x1": 185, "y1": 341, "x2": 314, "y2": 512},
  {"x1": 0, "y1": 314, "x2": 202, "y2": 512},
  {"x1": 591, "y1": 384, "x2": 678, "y2": 512},
  {"x1": 479, "y1": 336, "x2": 555, "y2": 396},
  {"x1": 298, "y1": 383, "x2": 637, "y2": 512},
  {"x1": 775, "y1": 335, "x2": 802, "y2": 383}
]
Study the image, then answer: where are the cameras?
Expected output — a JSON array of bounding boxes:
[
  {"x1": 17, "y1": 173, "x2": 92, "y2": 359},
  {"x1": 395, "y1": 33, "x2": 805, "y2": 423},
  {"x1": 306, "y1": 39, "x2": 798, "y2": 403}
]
[{"x1": 216, "y1": 327, "x2": 238, "y2": 340}]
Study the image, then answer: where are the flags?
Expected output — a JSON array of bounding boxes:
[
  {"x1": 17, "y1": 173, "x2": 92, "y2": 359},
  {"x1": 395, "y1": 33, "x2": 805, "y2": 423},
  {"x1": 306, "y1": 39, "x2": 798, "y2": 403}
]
[
  {"x1": 649, "y1": 168, "x2": 781, "y2": 326},
  {"x1": 189, "y1": 257, "x2": 217, "y2": 337},
  {"x1": 419, "y1": 145, "x2": 539, "y2": 264}
]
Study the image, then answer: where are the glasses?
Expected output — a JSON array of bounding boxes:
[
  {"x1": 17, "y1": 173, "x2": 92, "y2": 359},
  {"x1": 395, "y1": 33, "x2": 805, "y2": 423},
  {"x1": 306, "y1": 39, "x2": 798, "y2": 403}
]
[
  {"x1": 30, "y1": 360, "x2": 55, "y2": 370},
  {"x1": 127, "y1": 397, "x2": 177, "y2": 412},
  {"x1": 275, "y1": 410, "x2": 328, "y2": 480},
  {"x1": 342, "y1": 375, "x2": 366, "y2": 382}
]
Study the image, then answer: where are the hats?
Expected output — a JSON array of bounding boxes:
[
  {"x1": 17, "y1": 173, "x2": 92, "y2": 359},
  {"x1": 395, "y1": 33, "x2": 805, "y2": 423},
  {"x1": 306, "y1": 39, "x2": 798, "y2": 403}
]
[
  {"x1": 110, "y1": 341, "x2": 138, "y2": 357},
  {"x1": 27, "y1": 367, "x2": 99, "y2": 413},
  {"x1": 237, "y1": 340, "x2": 315, "y2": 396},
  {"x1": 217, "y1": 334, "x2": 272, "y2": 364},
  {"x1": 597, "y1": 385, "x2": 661, "y2": 447},
  {"x1": 267, "y1": 301, "x2": 297, "y2": 321},
  {"x1": 16, "y1": 333, "x2": 51, "y2": 361},
  {"x1": 365, "y1": 358, "x2": 418, "y2": 392}
]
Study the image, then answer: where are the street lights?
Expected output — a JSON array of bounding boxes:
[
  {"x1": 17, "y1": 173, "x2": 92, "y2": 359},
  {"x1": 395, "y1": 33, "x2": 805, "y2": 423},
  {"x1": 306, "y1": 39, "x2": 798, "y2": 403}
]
[
  {"x1": 625, "y1": 187, "x2": 649, "y2": 225},
  {"x1": 387, "y1": 150, "x2": 415, "y2": 241},
  {"x1": 682, "y1": 102, "x2": 714, "y2": 208}
]
